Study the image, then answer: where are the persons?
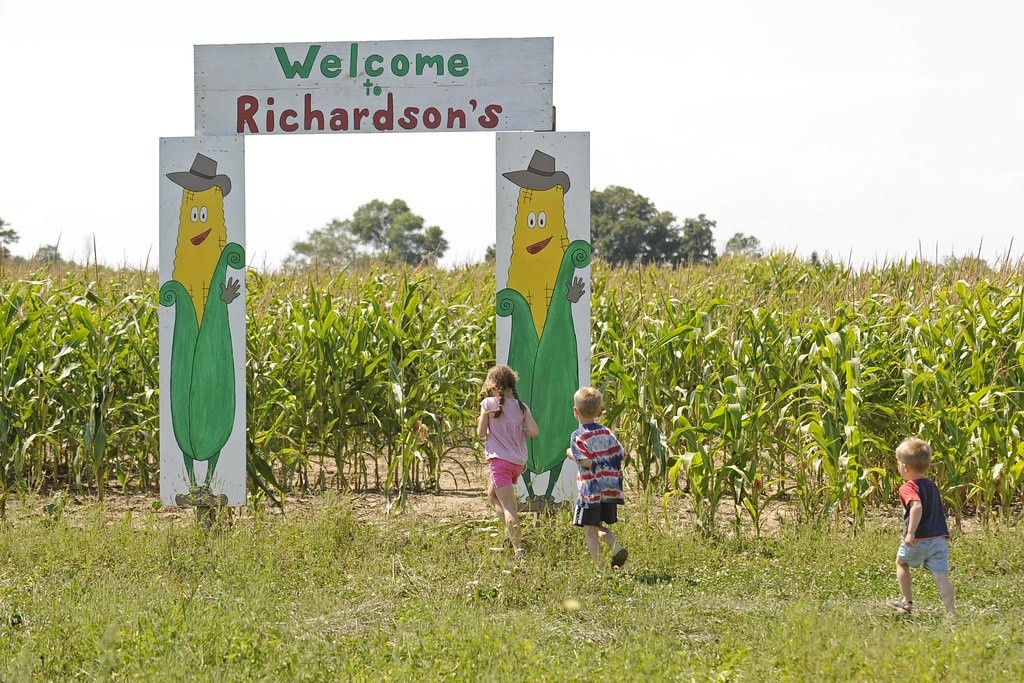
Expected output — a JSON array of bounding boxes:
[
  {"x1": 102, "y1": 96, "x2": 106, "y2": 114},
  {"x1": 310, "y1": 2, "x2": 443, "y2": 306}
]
[
  {"x1": 883, "y1": 437, "x2": 958, "y2": 626},
  {"x1": 565, "y1": 385, "x2": 630, "y2": 575},
  {"x1": 475, "y1": 362, "x2": 539, "y2": 563}
]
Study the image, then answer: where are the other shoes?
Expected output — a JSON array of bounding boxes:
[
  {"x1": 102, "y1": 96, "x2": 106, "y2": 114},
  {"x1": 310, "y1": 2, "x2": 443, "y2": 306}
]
[{"x1": 610, "y1": 549, "x2": 628, "y2": 571}]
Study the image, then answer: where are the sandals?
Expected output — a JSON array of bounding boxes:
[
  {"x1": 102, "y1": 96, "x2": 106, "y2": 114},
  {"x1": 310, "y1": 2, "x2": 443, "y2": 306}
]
[{"x1": 886, "y1": 599, "x2": 913, "y2": 615}]
